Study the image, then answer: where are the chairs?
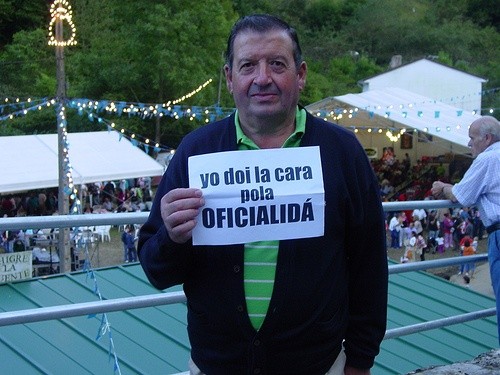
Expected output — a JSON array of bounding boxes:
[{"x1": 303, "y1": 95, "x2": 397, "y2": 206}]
[{"x1": 25, "y1": 225, "x2": 111, "y2": 248}]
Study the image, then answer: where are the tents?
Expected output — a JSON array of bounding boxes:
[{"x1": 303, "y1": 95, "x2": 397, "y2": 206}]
[
  {"x1": 304, "y1": 88, "x2": 483, "y2": 158},
  {"x1": 0, "y1": 130, "x2": 167, "y2": 217}
]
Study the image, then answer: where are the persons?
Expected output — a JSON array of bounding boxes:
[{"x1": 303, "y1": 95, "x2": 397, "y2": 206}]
[
  {"x1": 137, "y1": 16, "x2": 389, "y2": 375},
  {"x1": 431, "y1": 115, "x2": 500, "y2": 355},
  {"x1": 0, "y1": 176, "x2": 156, "y2": 285},
  {"x1": 370, "y1": 149, "x2": 488, "y2": 279}
]
